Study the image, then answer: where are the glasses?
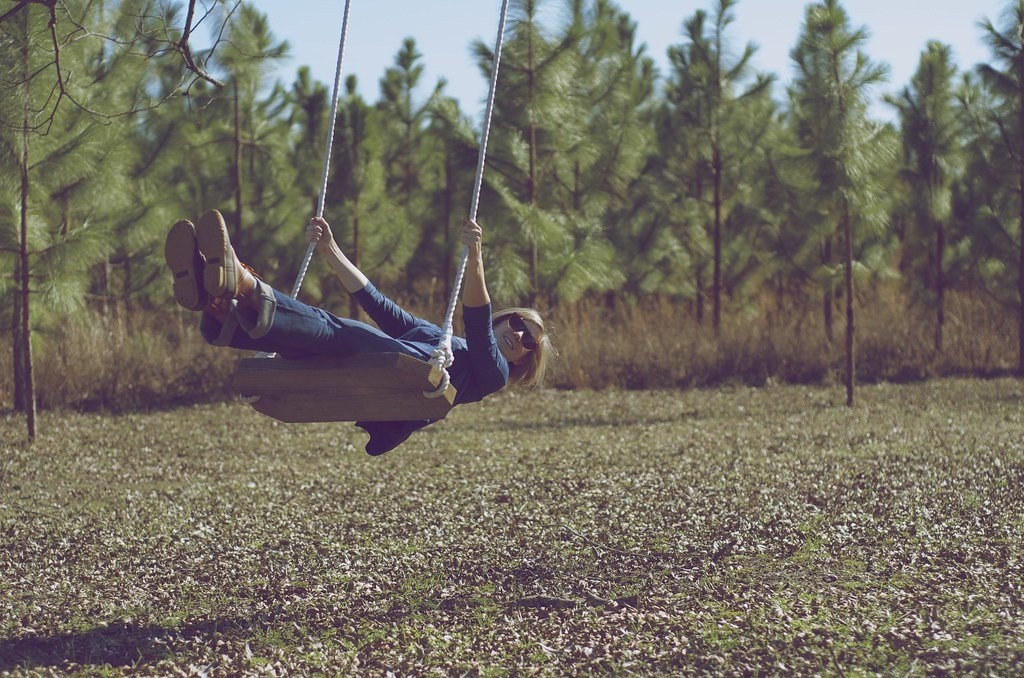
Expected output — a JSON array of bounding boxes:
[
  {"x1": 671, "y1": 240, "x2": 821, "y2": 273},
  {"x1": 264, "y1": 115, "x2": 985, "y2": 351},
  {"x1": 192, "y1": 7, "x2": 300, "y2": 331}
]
[{"x1": 509, "y1": 312, "x2": 538, "y2": 350}]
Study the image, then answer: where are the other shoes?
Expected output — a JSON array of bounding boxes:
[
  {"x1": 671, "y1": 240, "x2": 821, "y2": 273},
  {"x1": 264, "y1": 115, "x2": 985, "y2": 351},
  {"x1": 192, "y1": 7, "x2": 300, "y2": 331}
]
[{"x1": 165, "y1": 209, "x2": 266, "y2": 312}]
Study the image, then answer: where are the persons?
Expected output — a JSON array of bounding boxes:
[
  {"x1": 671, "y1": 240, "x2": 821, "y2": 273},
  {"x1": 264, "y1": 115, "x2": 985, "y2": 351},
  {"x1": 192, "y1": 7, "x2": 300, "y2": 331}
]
[{"x1": 164, "y1": 209, "x2": 559, "y2": 457}]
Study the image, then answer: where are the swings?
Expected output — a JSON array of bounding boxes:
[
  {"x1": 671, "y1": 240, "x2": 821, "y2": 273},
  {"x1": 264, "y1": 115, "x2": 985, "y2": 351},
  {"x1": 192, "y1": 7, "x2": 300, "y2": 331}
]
[{"x1": 231, "y1": 0, "x2": 514, "y2": 425}]
